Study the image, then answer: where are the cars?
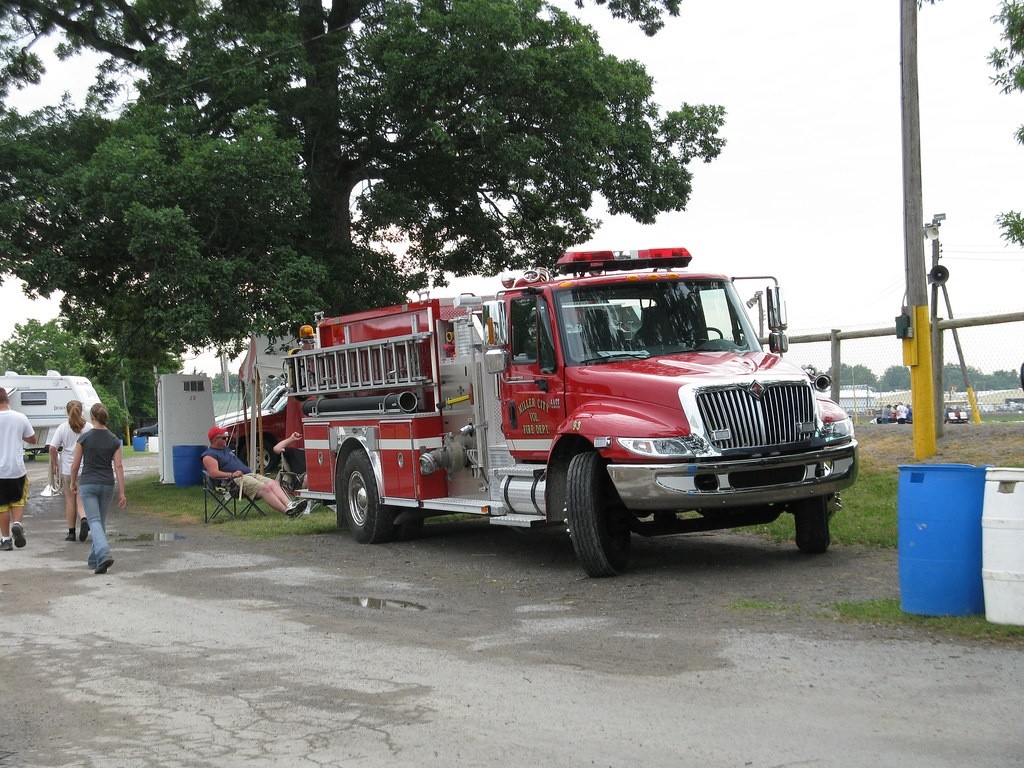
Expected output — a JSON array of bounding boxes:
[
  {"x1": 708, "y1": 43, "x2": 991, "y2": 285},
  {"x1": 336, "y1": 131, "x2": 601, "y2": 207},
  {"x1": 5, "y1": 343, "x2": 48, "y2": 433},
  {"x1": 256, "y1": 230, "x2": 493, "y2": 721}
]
[
  {"x1": 133, "y1": 422, "x2": 158, "y2": 437},
  {"x1": 214, "y1": 385, "x2": 288, "y2": 472}
]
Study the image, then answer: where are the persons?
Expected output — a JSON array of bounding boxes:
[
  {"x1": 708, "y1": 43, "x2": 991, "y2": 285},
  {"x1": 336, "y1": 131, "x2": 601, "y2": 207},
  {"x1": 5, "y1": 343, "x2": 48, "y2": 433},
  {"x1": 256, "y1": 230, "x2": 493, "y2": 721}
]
[
  {"x1": 200, "y1": 427, "x2": 307, "y2": 520},
  {"x1": 273, "y1": 432, "x2": 337, "y2": 514},
  {"x1": 882, "y1": 402, "x2": 913, "y2": 424},
  {"x1": 49, "y1": 400, "x2": 94, "y2": 542},
  {"x1": 0, "y1": 388, "x2": 36, "y2": 551},
  {"x1": 69, "y1": 403, "x2": 126, "y2": 573}
]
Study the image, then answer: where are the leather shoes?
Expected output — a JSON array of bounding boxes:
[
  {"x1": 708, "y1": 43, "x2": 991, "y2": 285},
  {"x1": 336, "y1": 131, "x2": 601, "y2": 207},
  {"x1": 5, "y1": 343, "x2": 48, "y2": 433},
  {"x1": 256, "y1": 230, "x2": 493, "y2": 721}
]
[
  {"x1": 65, "y1": 533, "x2": 76, "y2": 541},
  {"x1": 78, "y1": 519, "x2": 89, "y2": 542}
]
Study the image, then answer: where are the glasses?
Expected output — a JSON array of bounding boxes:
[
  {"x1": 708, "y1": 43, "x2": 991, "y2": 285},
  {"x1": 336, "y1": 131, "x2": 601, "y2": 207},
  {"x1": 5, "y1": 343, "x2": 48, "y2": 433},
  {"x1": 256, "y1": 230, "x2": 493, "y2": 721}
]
[{"x1": 216, "y1": 436, "x2": 227, "y2": 440}]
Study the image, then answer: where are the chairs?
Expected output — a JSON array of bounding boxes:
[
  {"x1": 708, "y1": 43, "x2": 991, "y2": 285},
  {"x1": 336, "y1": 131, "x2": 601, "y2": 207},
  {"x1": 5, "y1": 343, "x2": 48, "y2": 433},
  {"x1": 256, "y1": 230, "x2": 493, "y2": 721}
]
[
  {"x1": 640, "y1": 306, "x2": 676, "y2": 346},
  {"x1": 579, "y1": 309, "x2": 627, "y2": 360},
  {"x1": 202, "y1": 468, "x2": 267, "y2": 523},
  {"x1": 960, "y1": 412, "x2": 968, "y2": 422},
  {"x1": 947, "y1": 412, "x2": 958, "y2": 422},
  {"x1": 279, "y1": 451, "x2": 323, "y2": 513}
]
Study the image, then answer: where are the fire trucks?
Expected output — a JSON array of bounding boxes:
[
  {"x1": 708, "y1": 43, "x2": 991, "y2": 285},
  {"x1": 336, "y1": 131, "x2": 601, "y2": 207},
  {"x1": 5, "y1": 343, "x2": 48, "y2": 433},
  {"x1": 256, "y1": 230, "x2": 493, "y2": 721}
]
[{"x1": 283, "y1": 248, "x2": 859, "y2": 579}]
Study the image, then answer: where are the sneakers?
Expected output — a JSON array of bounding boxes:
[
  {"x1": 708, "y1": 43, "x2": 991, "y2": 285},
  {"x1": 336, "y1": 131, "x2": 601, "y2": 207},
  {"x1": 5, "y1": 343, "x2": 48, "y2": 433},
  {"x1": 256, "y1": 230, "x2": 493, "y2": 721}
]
[
  {"x1": 93, "y1": 558, "x2": 115, "y2": 574},
  {"x1": 285, "y1": 499, "x2": 308, "y2": 521},
  {"x1": 87, "y1": 548, "x2": 97, "y2": 569},
  {"x1": 0, "y1": 538, "x2": 13, "y2": 551},
  {"x1": 11, "y1": 523, "x2": 26, "y2": 548}
]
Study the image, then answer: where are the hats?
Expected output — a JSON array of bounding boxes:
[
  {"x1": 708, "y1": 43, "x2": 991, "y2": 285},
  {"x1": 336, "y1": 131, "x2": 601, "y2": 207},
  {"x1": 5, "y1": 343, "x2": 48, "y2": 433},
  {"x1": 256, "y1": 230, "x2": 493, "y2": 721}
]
[{"x1": 207, "y1": 427, "x2": 227, "y2": 439}]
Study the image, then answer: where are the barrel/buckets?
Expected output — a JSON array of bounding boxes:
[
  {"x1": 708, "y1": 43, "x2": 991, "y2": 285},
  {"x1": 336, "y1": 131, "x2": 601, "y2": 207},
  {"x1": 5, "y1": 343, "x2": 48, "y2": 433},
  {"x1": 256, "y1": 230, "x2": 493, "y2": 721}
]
[
  {"x1": 149, "y1": 437, "x2": 158, "y2": 452},
  {"x1": 172, "y1": 445, "x2": 208, "y2": 488},
  {"x1": 895, "y1": 463, "x2": 993, "y2": 617},
  {"x1": 981, "y1": 467, "x2": 1024, "y2": 626},
  {"x1": 132, "y1": 437, "x2": 146, "y2": 451}
]
